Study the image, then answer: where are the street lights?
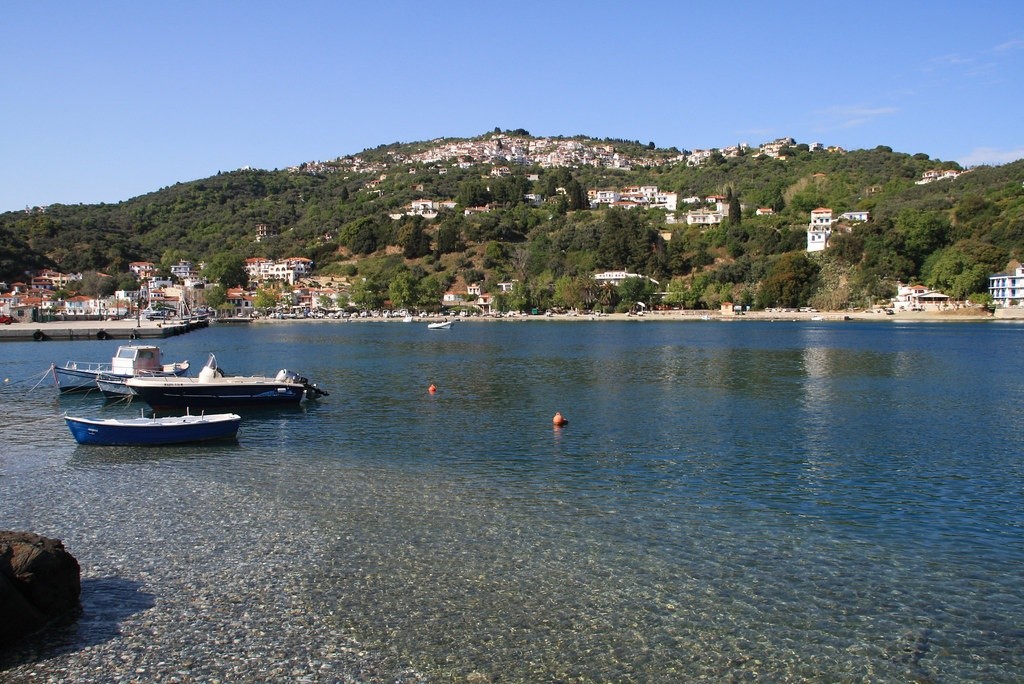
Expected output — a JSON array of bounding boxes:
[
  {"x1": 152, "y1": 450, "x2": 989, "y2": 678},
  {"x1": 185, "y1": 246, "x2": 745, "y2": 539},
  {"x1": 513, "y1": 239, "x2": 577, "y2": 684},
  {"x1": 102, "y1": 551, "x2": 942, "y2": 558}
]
[{"x1": 39, "y1": 289, "x2": 44, "y2": 323}]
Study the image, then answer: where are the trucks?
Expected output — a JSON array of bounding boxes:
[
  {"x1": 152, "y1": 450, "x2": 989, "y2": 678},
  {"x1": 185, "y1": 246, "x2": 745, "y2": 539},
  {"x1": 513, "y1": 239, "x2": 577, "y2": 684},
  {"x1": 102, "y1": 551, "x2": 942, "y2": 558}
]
[{"x1": 146, "y1": 307, "x2": 178, "y2": 321}]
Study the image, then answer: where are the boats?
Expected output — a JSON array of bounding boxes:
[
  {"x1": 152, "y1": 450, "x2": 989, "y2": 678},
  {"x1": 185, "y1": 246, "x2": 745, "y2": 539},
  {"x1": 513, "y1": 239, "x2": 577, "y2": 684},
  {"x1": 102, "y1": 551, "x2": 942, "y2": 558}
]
[
  {"x1": 63, "y1": 406, "x2": 242, "y2": 446},
  {"x1": 428, "y1": 322, "x2": 454, "y2": 330},
  {"x1": 51, "y1": 341, "x2": 191, "y2": 394},
  {"x1": 183, "y1": 314, "x2": 208, "y2": 320},
  {"x1": 811, "y1": 315, "x2": 824, "y2": 321},
  {"x1": 217, "y1": 318, "x2": 254, "y2": 324},
  {"x1": 95, "y1": 367, "x2": 225, "y2": 400},
  {"x1": 126, "y1": 353, "x2": 329, "y2": 410}
]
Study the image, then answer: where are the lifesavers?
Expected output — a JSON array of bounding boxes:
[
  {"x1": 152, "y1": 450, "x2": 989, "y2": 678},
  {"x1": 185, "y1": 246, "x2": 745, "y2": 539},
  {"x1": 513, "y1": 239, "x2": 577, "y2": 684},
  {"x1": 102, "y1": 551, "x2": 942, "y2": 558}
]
[
  {"x1": 128, "y1": 333, "x2": 135, "y2": 339},
  {"x1": 97, "y1": 331, "x2": 106, "y2": 340},
  {"x1": 33, "y1": 331, "x2": 44, "y2": 341}
]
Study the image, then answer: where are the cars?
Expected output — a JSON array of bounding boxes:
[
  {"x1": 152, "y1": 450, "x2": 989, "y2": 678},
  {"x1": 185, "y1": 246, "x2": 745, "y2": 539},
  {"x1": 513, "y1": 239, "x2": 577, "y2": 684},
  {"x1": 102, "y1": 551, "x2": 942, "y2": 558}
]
[
  {"x1": 270, "y1": 305, "x2": 554, "y2": 319},
  {"x1": 0, "y1": 313, "x2": 14, "y2": 325}
]
